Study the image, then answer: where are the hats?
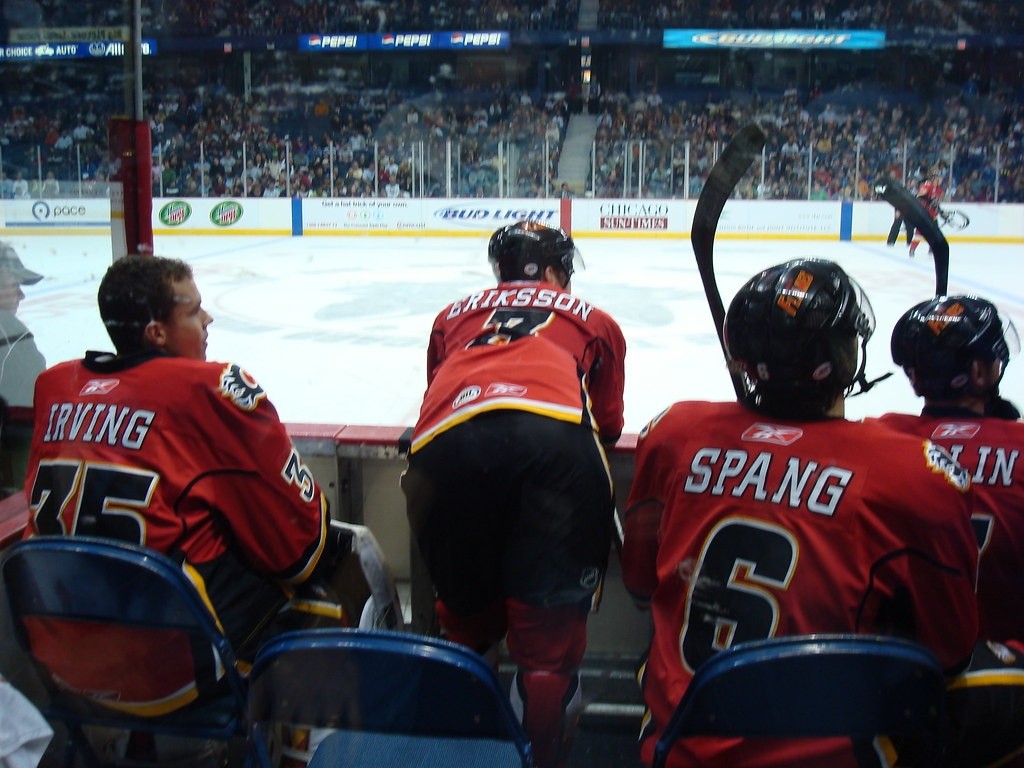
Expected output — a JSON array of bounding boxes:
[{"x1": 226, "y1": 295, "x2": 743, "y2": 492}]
[{"x1": 0, "y1": 242, "x2": 44, "y2": 286}]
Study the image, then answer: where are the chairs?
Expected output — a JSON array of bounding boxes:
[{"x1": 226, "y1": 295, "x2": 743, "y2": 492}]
[
  {"x1": 653, "y1": 632, "x2": 948, "y2": 768},
  {"x1": 244, "y1": 629, "x2": 537, "y2": 768},
  {"x1": 0, "y1": 534, "x2": 274, "y2": 768}
]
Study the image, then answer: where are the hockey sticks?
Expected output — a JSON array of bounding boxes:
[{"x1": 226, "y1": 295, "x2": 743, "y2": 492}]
[
  {"x1": 875, "y1": 176, "x2": 949, "y2": 297},
  {"x1": 613, "y1": 509, "x2": 647, "y2": 613},
  {"x1": 690, "y1": 116, "x2": 770, "y2": 404}
]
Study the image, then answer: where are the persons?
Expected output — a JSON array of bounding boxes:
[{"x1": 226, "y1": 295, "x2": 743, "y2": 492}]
[
  {"x1": 401, "y1": 221, "x2": 626, "y2": 768},
  {"x1": 0, "y1": 1, "x2": 1024, "y2": 768}
]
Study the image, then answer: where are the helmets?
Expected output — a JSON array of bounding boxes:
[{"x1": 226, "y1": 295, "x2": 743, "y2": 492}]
[
  {"x1": 488, "y1": 221, "x2": 575, "y2": 283},
  {"x1": 891, "y1": 294, "x2": 1010, "y2": 395},
  {"x1": 723, "y1": 258, "x2": 865, "y2": 422}
]
